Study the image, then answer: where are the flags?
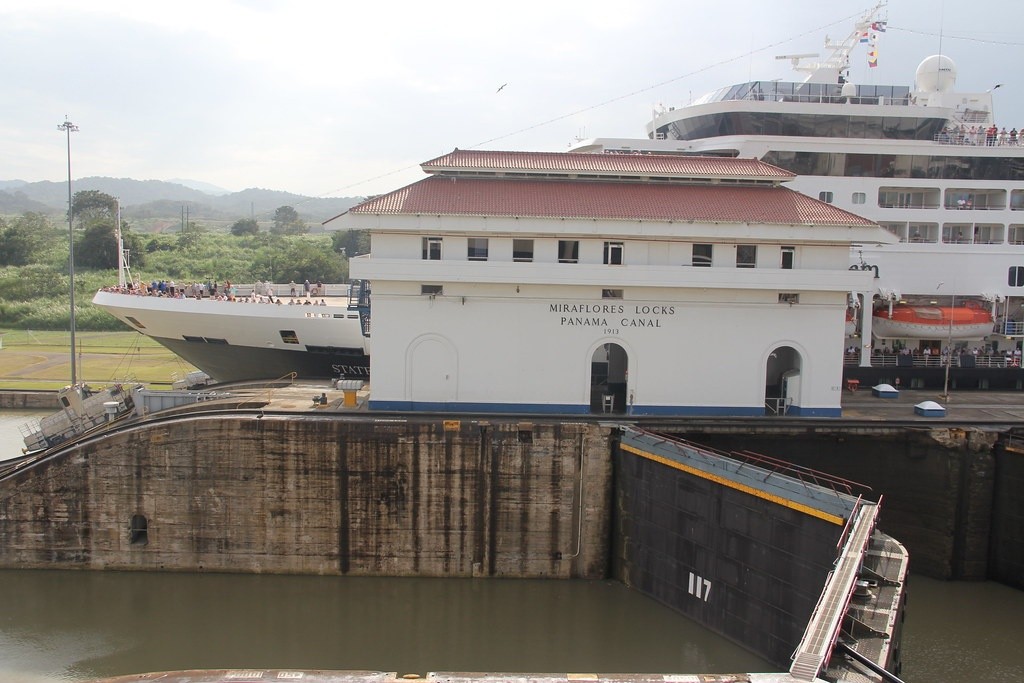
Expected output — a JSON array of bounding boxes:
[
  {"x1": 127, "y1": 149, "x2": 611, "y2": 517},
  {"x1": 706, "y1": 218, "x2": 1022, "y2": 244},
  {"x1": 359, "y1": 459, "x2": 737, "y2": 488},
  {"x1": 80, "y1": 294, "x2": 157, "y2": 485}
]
[{"x1": 860, "y1": 32, "x2": 878, "y2": 68}]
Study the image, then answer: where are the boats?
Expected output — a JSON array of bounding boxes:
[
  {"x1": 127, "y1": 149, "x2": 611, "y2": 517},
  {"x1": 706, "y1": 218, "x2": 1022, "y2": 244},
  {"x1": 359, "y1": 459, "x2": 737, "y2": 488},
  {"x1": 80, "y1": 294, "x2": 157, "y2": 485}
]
[{"x1": 92, "y1": 3, "x2": 1024, "y2": 384}]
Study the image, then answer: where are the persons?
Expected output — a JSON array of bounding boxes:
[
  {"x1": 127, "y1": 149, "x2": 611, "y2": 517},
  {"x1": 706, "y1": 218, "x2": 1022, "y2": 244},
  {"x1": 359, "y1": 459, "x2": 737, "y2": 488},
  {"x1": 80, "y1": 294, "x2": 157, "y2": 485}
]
[
  {"x1": 940, "y1": 121, "x2": 1024, "y2": 148},
  {"x1": 100, "y1": 274, "x2": 238, "y2": 304},
  {"x1": 845, "y1": 339, "x2": 1022, "y2": 370},
  {"x1": 318, "y1": 393, "x2": 327, "y2": 405},
  {"x1": 880, "y1": 156, "x2": 944, "y2": 180},
  {"x1": 903, "y1": 91, "x2": 917, "y2": 105},
  {"x1": 895, "y1": 377, "x2": 900, "y2": 390},
  {"x1": 237, "y1": 279, "x2": 327, "y2": 306},
  {"x1": 956, "y1": 195, "x2": 974, "y2": 211},
  {"x1": 912, "y1": 226, "x2": 981, "y2": 244},
  {"x1": 752, "y1": 88, "x2": 764, "y2": 101}
]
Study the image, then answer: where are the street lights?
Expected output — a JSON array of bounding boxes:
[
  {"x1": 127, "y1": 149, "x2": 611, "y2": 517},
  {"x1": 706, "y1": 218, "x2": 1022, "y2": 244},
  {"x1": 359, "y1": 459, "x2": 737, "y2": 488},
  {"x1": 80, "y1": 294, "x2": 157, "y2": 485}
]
[{"x1": 56, "y1": 114, "x2": 80, "y2": 385}]
